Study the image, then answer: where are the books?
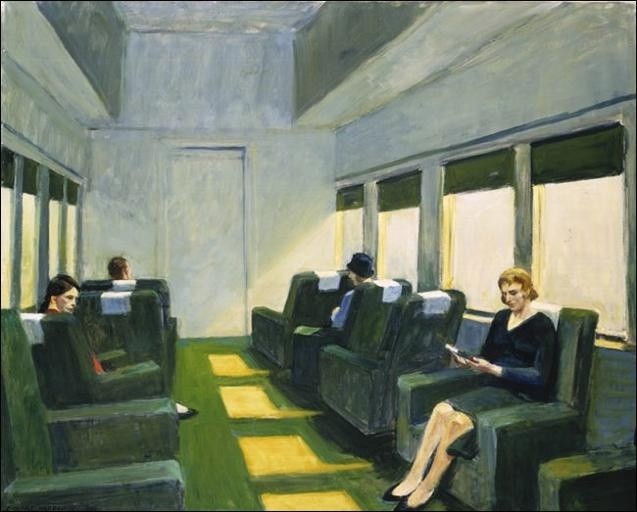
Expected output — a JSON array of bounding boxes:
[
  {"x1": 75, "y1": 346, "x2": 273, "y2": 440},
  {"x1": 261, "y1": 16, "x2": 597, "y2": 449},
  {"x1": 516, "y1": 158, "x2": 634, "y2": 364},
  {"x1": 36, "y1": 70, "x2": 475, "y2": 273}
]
[{"x1": 445, "y1": 343, "x2": 473, "y2": 361}]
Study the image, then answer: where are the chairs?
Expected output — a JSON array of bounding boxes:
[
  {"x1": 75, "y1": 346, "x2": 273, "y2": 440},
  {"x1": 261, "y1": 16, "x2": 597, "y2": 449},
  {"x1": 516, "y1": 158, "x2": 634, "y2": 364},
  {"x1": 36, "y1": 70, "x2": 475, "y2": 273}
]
[
  {"x1": 247, "y1": 264, "x2": 636, "y2": 511},
  {"x1": 0, "y1": 276, "x2": 185, "y2": 511}
]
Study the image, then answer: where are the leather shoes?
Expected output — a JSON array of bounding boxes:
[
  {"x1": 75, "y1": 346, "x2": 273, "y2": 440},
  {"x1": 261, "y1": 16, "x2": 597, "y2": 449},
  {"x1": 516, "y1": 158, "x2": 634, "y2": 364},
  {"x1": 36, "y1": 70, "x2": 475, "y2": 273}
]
[
  {"x1": 380, "y1": 479, "x2": 436, "y2": 511},
  {"x1": 176, "y1": 405, "x2": 198, "y2": 420}
]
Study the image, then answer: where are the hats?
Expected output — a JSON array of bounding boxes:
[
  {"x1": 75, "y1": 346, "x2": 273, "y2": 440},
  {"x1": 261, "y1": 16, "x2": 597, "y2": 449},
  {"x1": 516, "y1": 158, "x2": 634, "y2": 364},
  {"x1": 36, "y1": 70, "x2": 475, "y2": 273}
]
[{"x1": 346, "y1": 251, "x2": 375, "y2": 279}]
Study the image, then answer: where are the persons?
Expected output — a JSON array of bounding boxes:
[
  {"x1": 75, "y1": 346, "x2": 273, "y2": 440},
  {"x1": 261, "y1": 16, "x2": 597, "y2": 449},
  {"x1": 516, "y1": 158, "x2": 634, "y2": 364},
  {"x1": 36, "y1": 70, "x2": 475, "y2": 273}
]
[
  {"x1": 108, "y1": 256, "x2": 133, "y2": 281},
  {"x1": 331, "y1": 253, "x2": 376, "y2": 332},
  {"x1": 378, "y1": 267, "x2": 558, "y2": 511},
  {"x1": 36, "y1": 272, "x2": 201, "y2": 421}
]
